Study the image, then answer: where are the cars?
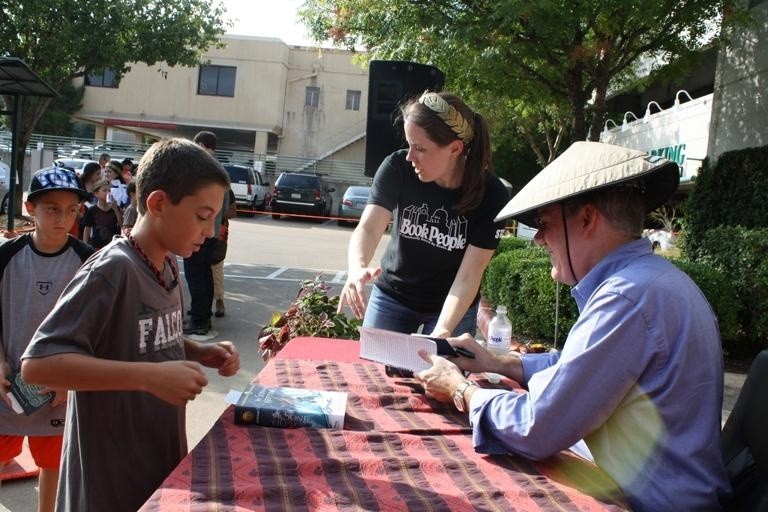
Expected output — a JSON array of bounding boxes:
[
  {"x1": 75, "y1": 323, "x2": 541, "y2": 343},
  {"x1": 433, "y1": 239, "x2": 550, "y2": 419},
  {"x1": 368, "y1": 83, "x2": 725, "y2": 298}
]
[
  {"x1": 53, "y1": 158, "x2": 98, "y2": 177},
  {"x1": 337, "y1": 185, "x2": 389, "y2": 231},
  {"x1": 0, "y1": 156, "x2": 19, "y2": 214}
]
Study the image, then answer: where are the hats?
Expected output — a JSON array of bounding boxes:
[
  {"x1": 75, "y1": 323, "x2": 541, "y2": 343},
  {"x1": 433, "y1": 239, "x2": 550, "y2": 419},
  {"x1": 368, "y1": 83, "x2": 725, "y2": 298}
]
[
  {"x1": 28, "y1": 167, "x2": 90, "y2": 203},
  {"x1": 493, "y1": 141, "x2": 682, "y2": 223}
]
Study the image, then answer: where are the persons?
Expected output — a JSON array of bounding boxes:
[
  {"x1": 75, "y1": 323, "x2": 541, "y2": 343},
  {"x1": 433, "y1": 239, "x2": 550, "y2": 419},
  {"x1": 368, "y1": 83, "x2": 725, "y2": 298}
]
[
  {"x1": 0, "y1": 130, "x2": 242, "y2": 511},
  {"x1": 413, "y1": 140, "x2": 737, "y2": 512},
  {"x1": 336, "y1": 91, "x2": 511, "y2": 341}
]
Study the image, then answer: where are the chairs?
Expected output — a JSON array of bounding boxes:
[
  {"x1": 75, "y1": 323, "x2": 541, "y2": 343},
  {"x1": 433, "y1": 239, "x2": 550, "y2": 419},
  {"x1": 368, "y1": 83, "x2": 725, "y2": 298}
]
[{"x1": 721, "y1": 350, "x2": 767, "y2": 512}]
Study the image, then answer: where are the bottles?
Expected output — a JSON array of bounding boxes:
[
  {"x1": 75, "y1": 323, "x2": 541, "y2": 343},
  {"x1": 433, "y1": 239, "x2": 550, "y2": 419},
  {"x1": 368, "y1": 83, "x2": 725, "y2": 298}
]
[{"x1": 486, "y1": 304, "x2": 514, "y2": 379}]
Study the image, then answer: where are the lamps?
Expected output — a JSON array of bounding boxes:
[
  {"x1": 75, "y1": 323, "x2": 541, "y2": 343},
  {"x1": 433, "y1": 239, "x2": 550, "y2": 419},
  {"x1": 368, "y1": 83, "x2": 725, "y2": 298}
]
[{"x1": 602, "y1": 90, "x2": 694, "y2": 138}]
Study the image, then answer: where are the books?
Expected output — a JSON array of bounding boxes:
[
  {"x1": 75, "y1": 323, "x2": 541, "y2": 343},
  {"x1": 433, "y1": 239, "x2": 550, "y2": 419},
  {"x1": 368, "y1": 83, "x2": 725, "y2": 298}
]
[{"x1": 234, "y1": 382, "x2": 349, "y2": 429}]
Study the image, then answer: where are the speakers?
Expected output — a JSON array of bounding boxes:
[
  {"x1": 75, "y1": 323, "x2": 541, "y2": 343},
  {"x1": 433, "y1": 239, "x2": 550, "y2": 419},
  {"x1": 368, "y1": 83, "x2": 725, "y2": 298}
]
[{"x1": 364, "y1": 59, "x2": 446, "y2": 177}]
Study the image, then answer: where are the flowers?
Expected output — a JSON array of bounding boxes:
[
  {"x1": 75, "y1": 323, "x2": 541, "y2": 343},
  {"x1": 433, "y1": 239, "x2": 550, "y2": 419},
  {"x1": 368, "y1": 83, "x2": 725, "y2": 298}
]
[{"x1": 257, "y1": 270, "x2": 362, "y2": 361}]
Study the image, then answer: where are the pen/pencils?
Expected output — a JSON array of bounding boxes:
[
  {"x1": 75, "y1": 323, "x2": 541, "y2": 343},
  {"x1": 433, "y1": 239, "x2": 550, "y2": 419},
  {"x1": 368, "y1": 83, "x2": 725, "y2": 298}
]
[{"x1": 452, "y1": 347, "x2": 474, "y2": 360}]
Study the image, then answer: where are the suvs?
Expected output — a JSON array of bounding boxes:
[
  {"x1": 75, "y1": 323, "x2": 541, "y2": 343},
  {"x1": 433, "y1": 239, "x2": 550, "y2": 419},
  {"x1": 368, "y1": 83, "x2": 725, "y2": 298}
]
[
  {"x1": 271, "y1": 169, "x2": 336, "y2": 223},
  {"x1": 222, "y1": 163, "x2": 270, "y2": 217}
]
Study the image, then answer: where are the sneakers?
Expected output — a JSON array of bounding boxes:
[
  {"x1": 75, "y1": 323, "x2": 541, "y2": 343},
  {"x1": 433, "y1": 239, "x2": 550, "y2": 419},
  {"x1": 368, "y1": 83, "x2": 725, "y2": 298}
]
[{"x1": 183, "y1": 307, "x2": 224, "y2": 335}]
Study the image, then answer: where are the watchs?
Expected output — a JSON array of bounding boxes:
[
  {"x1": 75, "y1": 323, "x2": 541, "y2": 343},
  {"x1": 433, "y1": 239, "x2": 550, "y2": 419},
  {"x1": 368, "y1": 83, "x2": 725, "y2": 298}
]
[{"x1": 453, "y1": 379, "x2": 477, "y2": 414}]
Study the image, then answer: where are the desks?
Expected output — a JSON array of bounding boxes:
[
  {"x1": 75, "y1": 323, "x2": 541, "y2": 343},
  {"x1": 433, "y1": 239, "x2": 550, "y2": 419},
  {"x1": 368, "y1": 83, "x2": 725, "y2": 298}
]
[{"x1": 134, "y1": 336, "x2": 632, "y2": 511}]
